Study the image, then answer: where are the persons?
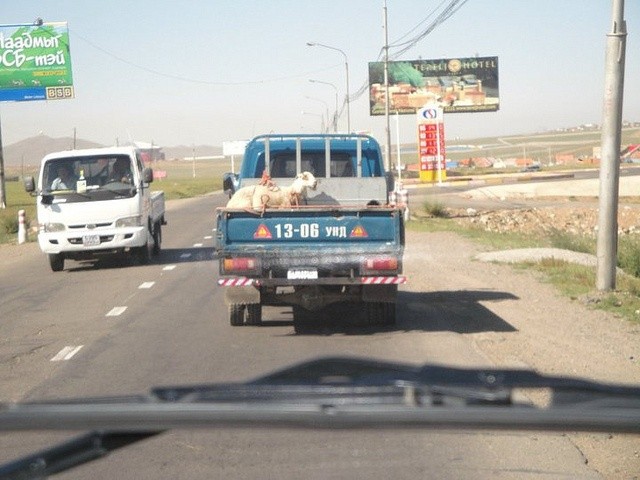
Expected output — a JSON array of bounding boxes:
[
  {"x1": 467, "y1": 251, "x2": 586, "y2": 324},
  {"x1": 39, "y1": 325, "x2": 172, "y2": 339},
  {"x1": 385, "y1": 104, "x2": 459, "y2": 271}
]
[
  {"x1": 106, "y1": 155, "x2": 132, "y2": 185},
  {"x1": 50, "y1": 165, "x2": 79, "y2": 192}
]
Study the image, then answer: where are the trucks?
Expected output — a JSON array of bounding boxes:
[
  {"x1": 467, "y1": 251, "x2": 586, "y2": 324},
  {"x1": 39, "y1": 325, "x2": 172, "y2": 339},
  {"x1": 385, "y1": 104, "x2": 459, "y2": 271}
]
[
  {"x1": 215, "y1": 134, "x2": 405, "y2": 325},
  {"x1": 24, "y1": 145, "x2": 167, "y2": 271}
]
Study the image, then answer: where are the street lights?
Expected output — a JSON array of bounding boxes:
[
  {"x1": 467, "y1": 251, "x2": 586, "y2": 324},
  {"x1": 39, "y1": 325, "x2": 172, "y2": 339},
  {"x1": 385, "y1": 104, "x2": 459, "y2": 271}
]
[
  {"x1": 307, "y1": 42, "x2": 350, "y2": 134},
  {"x1": 309, "y1": 80, "x2": 338, "y2": 133}
]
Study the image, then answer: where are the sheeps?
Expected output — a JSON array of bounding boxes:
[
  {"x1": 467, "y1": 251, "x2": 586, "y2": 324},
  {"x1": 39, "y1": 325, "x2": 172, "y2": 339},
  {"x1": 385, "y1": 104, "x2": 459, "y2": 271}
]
[{"x1": 226, "y1": 171, "x2": 317, "y2": 211}]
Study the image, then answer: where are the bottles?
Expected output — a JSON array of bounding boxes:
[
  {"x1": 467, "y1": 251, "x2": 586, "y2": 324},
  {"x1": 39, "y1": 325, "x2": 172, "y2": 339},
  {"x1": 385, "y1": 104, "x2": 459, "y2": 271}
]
[{"x1": 79, "y1": 170, "x2": 85, "y2": 181}]
